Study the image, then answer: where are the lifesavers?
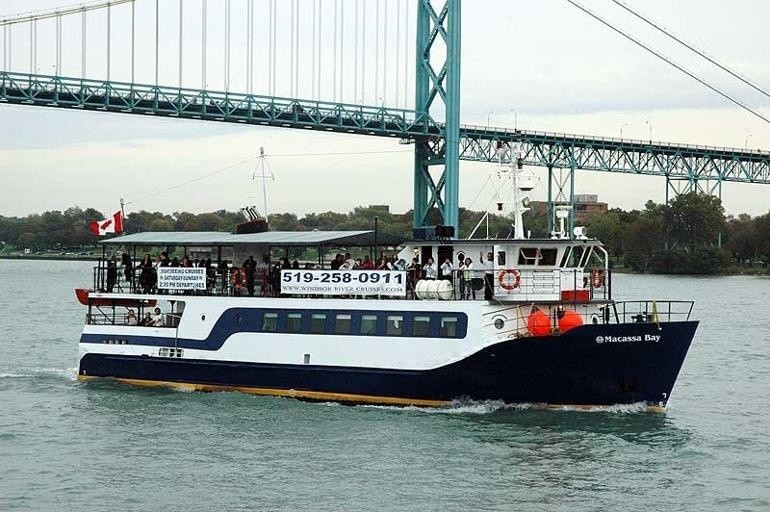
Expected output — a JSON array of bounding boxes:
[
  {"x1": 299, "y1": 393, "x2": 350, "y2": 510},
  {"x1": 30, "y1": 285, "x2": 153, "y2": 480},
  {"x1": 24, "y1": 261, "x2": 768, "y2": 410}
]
[
  {"x1": 591, "y1": 269, "x2": 602, "y2": 288},
  {"x1": 499, "y1": 270, "x2": 520, "y2": 289},
  {"x1": 232, "y1": 270, "x2": 247, "y2": 291}
]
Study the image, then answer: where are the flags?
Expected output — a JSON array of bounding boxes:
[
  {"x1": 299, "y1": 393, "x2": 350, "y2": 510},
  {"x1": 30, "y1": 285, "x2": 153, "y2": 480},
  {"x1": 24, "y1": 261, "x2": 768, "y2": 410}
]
[{"x1": 89, "y1": 209, "x2": 122, "y2": 238}]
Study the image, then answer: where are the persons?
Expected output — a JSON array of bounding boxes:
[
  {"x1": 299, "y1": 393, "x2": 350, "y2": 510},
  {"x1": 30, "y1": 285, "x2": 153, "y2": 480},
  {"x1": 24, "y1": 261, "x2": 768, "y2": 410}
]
[
  {"x1": 137, "y1": 311, "x2": 156, "y2": 327},
  {"x1": 124, "y1": 309, "x2": 139, "y2": 326},
  {"x1": 145, "y1": 307, "x2": 165, "y2": 327}
]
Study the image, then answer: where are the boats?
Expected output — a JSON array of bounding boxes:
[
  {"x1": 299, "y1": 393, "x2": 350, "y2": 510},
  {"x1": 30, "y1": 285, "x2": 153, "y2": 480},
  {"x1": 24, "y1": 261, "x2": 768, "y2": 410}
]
[{"x1": 78, "y1": 230, "x2": 698, "y2": 412}]
